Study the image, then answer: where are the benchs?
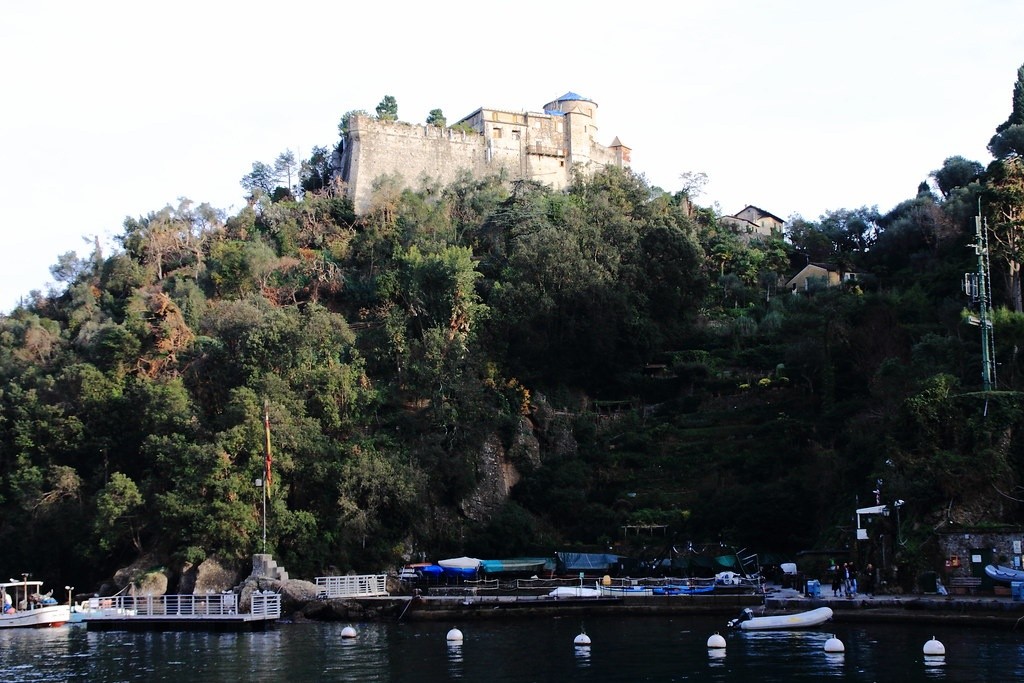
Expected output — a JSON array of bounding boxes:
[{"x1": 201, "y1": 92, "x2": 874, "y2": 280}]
[{"x1": 945, "y1": 577, "x2": 982, "y2": 597}]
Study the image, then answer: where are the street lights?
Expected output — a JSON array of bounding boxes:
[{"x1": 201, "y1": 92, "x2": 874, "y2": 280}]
[{"x1": 65, "y1": 586, "x2": 74, "y2": 610}]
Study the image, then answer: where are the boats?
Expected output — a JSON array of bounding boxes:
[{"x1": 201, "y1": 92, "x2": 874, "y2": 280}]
[
  {"x1": 397, "y1": 551, "x2": 770, "y2": 598},
  {"x1": 30, "y1": 593, "x2": 58, "y2": 606},
  {"x1": 984, "y1": 563, "x2": 1024, "y2": 584},
  {"x1": 0, "y1": 578, "x2": 70, "y2": 629},
  {"x1": 727, "y1": 603, "x2": 834, "y2": 632}
]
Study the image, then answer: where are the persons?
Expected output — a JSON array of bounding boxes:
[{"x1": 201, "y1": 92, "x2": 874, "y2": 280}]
[
  {"x1": 412, "y1": 584, "x2": 422, "y2": 610},
  {"x1": 831, "y1": 560, "x2": 875, "y2": 599}
]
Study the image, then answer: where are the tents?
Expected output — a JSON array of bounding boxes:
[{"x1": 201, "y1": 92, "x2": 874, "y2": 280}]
[{"x1": 422, "y1": 552, "x2": 628, "y2": 586}]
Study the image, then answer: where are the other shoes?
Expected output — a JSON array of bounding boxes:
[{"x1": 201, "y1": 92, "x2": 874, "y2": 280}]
[
  {"x1": 840, "y1": 594, "x2": 843, "y2": 597},
  {"x1": 834, "y1": 595, "x2": 838, "y2": 597}
]
